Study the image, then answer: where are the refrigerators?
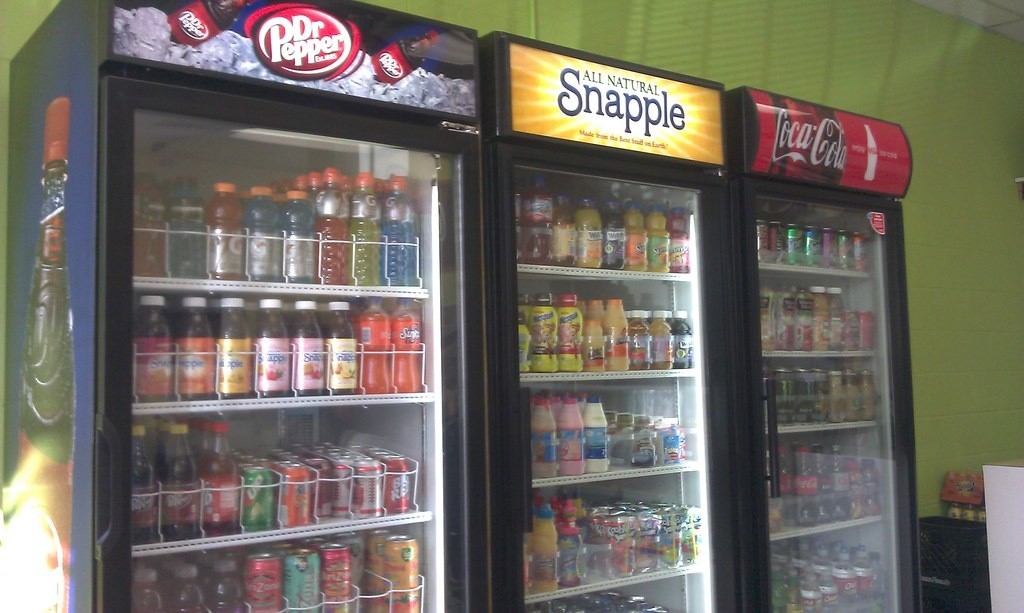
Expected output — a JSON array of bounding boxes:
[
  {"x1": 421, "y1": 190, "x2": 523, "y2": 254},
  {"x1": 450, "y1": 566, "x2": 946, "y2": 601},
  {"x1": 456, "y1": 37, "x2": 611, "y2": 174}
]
[
  {"x1": 0, "y1": 1, "x2": 480, "y2": 613},
  {"x1": 723, "y1": 85, "x2": 925, "y2": 613},
  {"x1": 480, "y1": 29, "x2": 739, "y2": 612}
]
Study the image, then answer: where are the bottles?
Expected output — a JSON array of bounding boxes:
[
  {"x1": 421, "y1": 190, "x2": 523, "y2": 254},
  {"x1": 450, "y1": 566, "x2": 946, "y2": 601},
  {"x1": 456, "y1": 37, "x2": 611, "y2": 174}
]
[
  {"x1": 167, "y1": 1, "x2": 252, "y2": 58},
  {"x1": 126, "y1": 413, "x2": 242, "y2": 546},
  {"x1": 757, "y1": 276, "x2": 845, "y2": 352},
  {"x1": 517, "y1": 291, "x2": 695, "y2": 372},
  {"x1": 133, "y1": 292, "x2": 424, "y2": 403},
  {"x1": 770, "y1": 537, "x2": 887, "y2": 613},
  {"x1": 862, "y1": 122, "x2": 879, "y2": 182},
  {"x1": 126, "y1": 551, "x2": 239, "y2": 612},
  {"x1": 523, "y1": 490, "x2": 586, "y2": 597},
  {"x1": 368, "y1": 30, "x2": 442, "y2": 94},
  {"x1": 132, "y1": 165, "x2": 420, "y2": 287},
  {"x1": 514, "y1": 172, "x2": 691, "y2": 272},
  {"x1": 10, "y1": 92, "x2": 73, "y2": 612},
  {"x1": 766, "y1": 440, "x2": 882, "y2": 532},
  {"x1": 527, "y1": 388, "x2": 610, "y2": 478}
]
